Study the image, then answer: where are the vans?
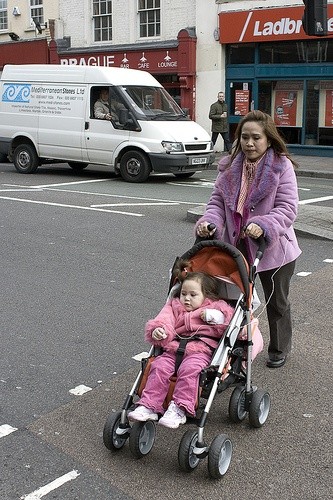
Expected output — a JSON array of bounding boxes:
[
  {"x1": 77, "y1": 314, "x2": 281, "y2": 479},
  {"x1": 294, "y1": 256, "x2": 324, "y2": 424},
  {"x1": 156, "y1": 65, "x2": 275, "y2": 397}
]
[{"x1": 1, "y1": 64, "x2": 215, "y2": 183}]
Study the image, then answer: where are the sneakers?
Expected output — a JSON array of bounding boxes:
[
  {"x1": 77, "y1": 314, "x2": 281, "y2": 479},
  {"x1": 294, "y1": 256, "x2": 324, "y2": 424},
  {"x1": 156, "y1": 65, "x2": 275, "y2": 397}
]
[
  {"x1": 127, "y1": 405, "x2": 159, "y2": 422},
  {"x1": 158, "y1": 401, "x2": 187, "y2": 429}
]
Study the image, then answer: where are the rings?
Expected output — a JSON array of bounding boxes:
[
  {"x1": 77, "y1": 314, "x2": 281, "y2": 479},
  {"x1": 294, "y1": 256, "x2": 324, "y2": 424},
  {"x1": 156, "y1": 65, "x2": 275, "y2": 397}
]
[{"x1": 199, "y1": 230, "x2": 201, "y2": 232}]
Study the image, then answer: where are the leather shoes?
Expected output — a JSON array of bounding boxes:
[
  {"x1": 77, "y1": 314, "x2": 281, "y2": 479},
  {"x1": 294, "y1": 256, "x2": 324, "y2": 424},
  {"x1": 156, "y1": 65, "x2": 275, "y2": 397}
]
[{"x1": 266, "y1": 359, "x2": 284, "y2": 368}]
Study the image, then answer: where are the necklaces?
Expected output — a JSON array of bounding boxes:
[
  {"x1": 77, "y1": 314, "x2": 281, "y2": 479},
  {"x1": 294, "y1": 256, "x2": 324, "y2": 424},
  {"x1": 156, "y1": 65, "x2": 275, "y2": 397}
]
[{"x1": 244, "y1": 158, "x2": 256, "y2": 186}]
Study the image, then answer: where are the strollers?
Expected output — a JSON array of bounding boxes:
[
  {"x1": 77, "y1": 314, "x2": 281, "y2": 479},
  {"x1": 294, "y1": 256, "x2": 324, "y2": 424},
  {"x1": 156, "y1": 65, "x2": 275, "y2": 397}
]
[{"x1": 103, "y1": 223, "x2": 271, "y2": 479}]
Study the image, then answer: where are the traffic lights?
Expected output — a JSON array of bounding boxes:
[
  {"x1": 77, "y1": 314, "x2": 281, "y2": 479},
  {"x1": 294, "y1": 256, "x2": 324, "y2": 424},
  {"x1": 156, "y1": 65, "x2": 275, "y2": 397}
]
[{"x1": 301, "y1": 0, "x2": 329, "y2": 37}]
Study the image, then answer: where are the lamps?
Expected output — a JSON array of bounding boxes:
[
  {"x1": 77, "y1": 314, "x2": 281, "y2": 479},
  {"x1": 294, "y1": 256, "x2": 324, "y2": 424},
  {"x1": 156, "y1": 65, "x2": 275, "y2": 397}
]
[
  {"x1": 140, "y1": 52, "x2": 147, "y2": 63},
  {"x1": 33, "y1": 18, "x2": 47, "y2": 35},
  {"x1": 164, "y1": 51, "x2": 171, "y2": 62},
  {"x1": 121, "y1": 53, "x2": 128, "y2": 64}
]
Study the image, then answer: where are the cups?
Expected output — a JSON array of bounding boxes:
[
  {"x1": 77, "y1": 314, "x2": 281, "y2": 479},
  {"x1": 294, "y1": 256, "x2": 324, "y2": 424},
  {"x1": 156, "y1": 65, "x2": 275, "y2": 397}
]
[{"x1": 223, "y1": 112, "x2": 227, "y2": 117}]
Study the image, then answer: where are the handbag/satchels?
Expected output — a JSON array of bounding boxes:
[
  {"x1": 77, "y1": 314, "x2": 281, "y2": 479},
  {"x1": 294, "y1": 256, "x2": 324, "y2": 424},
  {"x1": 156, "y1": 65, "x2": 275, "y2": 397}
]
[{"x1": 239, "y1": 319, "x2": 264, "y2": 369}]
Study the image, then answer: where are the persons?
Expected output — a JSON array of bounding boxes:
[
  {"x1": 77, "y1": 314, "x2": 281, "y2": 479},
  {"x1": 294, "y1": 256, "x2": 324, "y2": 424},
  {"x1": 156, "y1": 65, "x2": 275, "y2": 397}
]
[
  {"x1": 289, "y1": 92, "x2": 295, "y2": 99},
  {"x1": 209, "y1": 92, "x2": 231, "y2": 154},
  {"x1": 128, "y1": 270, "x2": 235, "y2": 428},
  {"x1": 194, "y1": 110, "x2": 303, "y2": 367},
  {"x1": 94, "y1": 90, "x2": 126, "y2": 121},
  {"x1": 277, "y1": 106, "x2": 283, "y2": 114}
]
